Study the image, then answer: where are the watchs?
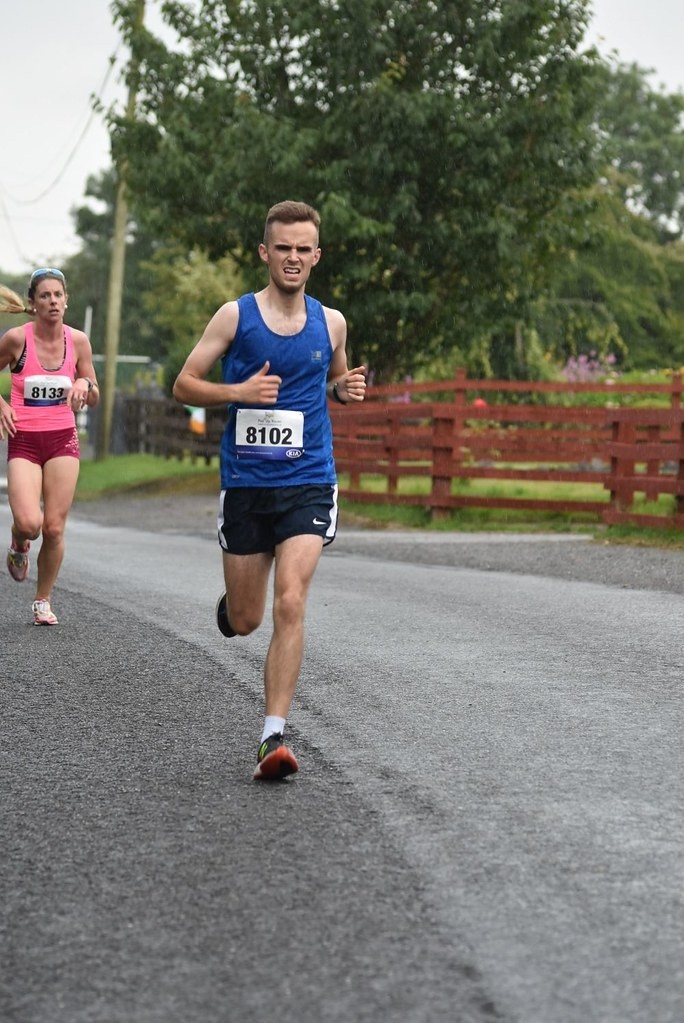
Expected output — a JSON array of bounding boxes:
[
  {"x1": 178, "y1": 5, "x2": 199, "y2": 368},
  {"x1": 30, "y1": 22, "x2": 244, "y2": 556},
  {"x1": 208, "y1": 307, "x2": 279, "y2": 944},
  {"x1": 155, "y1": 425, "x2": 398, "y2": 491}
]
[{"x1": 84, "y1": 377, "x2": 93, "y2": 395}]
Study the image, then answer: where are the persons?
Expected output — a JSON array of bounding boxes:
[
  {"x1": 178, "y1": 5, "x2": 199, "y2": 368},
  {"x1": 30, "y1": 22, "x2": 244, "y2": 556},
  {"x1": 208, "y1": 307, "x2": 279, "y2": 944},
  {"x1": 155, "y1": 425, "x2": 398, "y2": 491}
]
[
  {"x1": 171, "y1": 202, "x2": 367, "y2": 782},
  {"x1": 0, "y1": 269, "x2": 101, "y2": 627}
]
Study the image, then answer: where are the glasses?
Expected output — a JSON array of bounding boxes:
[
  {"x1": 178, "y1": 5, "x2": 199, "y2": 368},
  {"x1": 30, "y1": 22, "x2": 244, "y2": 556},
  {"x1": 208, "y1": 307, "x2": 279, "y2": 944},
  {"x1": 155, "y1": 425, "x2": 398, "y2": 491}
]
[{"x1": 31, "y1": 268, "x2": 65, "y2": 282}]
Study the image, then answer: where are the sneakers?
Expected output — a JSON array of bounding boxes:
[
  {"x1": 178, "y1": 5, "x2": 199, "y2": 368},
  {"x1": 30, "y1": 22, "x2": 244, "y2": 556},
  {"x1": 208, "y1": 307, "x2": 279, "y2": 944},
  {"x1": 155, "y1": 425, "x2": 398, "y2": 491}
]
[
  {"x1": 252, "y1": 733, "x2": 299, "y2": 781},
  {"x1": 32, "y1": 599, "x2": 59, "y2": 626},
  {"x1": 7, "y1": 544, "x2": 30, "y2": 582},
  {"x1": 215, "y1": 590, "x2": 239, "y2": 639}
]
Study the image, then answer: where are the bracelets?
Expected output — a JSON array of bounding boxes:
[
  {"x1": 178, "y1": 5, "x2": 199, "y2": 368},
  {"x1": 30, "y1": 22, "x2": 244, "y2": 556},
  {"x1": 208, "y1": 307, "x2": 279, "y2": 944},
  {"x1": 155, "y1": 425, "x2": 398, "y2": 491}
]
[{"x1": 333, "y1": 382, "x2": 348, "y2": 405}]
